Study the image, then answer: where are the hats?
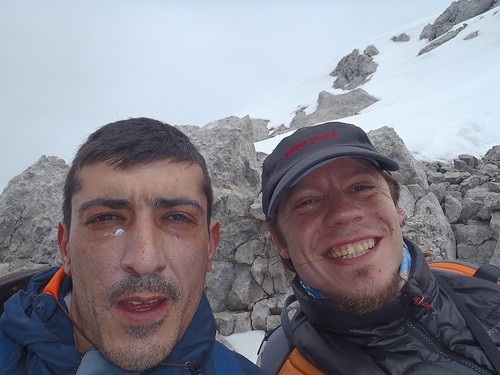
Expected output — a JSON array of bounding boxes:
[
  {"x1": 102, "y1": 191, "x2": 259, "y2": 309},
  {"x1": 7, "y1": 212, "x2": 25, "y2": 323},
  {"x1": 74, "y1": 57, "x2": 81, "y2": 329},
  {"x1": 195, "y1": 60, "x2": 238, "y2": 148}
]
[{"x1": 260, "y1": 122, "x2": 399, "y2": 223}]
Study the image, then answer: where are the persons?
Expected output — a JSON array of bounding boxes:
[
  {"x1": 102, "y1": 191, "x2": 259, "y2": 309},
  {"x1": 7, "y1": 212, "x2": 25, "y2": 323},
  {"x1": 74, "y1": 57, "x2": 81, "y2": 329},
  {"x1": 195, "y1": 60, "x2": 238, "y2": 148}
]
[
  {"x1": 257, "y1": 122, "x2": 500, "y2": 375},
  {"x1": 0, "y1": 118, "x2": 266, "y2": 375}
]
